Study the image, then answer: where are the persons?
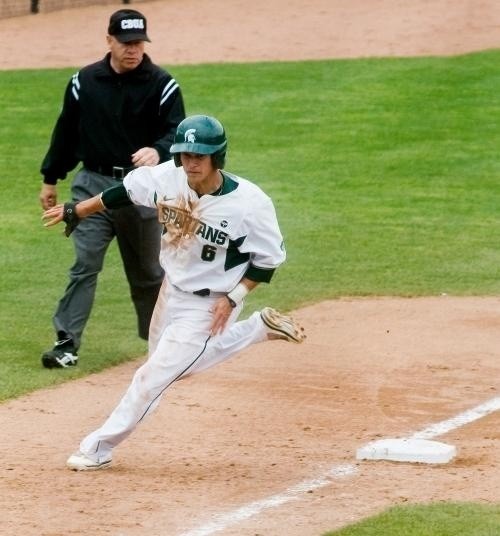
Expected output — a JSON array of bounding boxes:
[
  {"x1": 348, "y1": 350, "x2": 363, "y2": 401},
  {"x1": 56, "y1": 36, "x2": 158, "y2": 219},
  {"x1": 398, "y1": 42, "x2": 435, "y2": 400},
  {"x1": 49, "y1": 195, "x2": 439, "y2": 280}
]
[
  {"x1": 40, "y1": 114, "x2": 307, "y2": 472},
  {"x1": 41, "y1": 8, "x2": 186, "y2": 368}
]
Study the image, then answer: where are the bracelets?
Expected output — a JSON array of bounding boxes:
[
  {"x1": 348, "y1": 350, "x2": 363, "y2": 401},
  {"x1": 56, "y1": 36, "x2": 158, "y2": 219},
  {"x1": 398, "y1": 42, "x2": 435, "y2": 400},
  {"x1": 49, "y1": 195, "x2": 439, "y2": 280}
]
[{"x1": 227, "y1": 282, "x2": 250, "y2": 307}]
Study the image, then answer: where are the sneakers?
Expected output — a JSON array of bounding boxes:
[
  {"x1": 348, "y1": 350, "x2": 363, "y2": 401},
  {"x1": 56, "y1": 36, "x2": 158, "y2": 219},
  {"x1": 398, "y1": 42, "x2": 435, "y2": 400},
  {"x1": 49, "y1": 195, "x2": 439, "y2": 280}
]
[
  {"x1": 41, "y1": 337, "x2": 78, "y2": 367},
  {"x1": 66, "y1": 453, "x2": 113, "y2": 472},
  {"x1": 260, "y1": 307, "x2": 306, "y2": 344}
]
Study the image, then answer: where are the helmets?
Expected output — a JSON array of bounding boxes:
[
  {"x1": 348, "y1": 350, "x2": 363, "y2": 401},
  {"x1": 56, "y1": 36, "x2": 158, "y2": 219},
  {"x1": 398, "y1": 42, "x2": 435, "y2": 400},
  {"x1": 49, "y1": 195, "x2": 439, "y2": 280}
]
[{"x1": 170, "y1": 115, "x2": 226, "y2": 171}]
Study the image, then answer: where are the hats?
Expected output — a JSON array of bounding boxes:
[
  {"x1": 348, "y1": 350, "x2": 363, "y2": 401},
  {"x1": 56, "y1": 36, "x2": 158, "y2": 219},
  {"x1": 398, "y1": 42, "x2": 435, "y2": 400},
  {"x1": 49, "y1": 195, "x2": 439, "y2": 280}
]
[{"x1": 107, "y1": 10, "x2": 151, "y2": 44}]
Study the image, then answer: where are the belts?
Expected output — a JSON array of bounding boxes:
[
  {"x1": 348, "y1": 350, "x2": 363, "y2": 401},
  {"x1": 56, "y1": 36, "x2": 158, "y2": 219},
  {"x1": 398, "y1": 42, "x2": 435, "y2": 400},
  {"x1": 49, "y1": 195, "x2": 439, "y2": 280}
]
[
  {"x1": 195, "y1": 290, "x2": 211, "y2": 297},
  {"x1": 84, "y1": 162, "x2": 132, "y2": 180}
]
